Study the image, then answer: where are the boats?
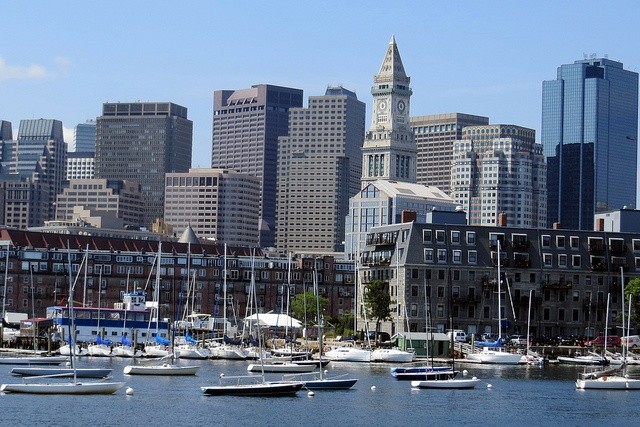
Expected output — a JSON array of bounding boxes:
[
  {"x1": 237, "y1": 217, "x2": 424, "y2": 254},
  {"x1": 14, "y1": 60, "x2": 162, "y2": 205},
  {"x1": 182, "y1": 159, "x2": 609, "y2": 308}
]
[
  {"x1": 262, "y1": 379, "x2": 358, "y2": 389},
  {"x1": 0, "y1": 357, "x2": 68, "y2": 364},
  {"x1": 123, "y1": 365, "x2": 200, "y2": 375},
  {"x1": 394, "y1": 370, "x2": 458, "y2": 377},
  {"x1": 134, "y1": 353, "x2": 173, "y2": 366},
  {"x1": 411, "y1": 378, "x2": 480, "y2": 388},
  {"x1": 576, "y1": 377, "x2": 640, "y2": 389},
  {"x1": 557, "y1": 356, "x2": 609, "y2": 364},
  {"x1": 1, "y1": 377, "x2": 126, "y2": 393},
  {"x1": 392, "y1": 367, "x2": 452, "y2": 373},
  {"x1": 11, "y1": 368, "x2": 113, "y2": 378},
  {"x1": 200, "y1": 382, "x2": 303, "y2": 394}
]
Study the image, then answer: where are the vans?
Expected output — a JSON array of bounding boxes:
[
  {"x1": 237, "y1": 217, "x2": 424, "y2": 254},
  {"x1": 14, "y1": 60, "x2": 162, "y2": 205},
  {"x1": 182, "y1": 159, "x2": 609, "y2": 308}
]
[
  {"x1": 620, "y1": 336, "x2": 640, "y2": 348},
  {"x1": 509, "y1": 335, "x2": 527, "y2": 346}
]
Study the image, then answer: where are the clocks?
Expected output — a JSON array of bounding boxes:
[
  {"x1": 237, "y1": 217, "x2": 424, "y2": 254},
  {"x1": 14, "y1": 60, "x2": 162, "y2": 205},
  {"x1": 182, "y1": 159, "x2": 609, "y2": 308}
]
[
  {"x1": 378, "y1": 100, "x2": 387, "y2": 110},
  {"x1": 397, "y1": 99, "x2": 406, "y2": 113}
]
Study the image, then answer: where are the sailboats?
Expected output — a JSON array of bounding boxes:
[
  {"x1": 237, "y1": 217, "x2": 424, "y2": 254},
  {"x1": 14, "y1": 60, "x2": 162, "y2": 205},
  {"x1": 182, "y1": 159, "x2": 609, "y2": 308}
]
[
  {"x1": 175, "y1": 242, "x2": 208, "y2": 358},
  {"x1": 261, "y1": 359, "x2": 329, "y2": 367},
  {"x1": 141, "y1": 243, "x2": 180, "y2": 356},
  {"x1": 59, "y1": 274, "x2": 89, "y2": 356},
  {"x1": 248, "y1": 365, "x2": 315, "y2": 372},
  {"x1": 371, "y1": 242, "x2": 412, "y2": 362},
  {"x1": 199, "y1": 242, "x2": 245, "y2": 359},
  {"x1": 468, "y1": 240, "x2": 522, "y2": 365},
  {"x1": 311, "y1": 246, "x2": 375, "y2": 362},
  {"x1": 271, "y1": 251, "x2": 311, "y2": 359},
  {"x1": 88, "y1": 268, "x2": 116, "y2": 356},
  {"x1": 112, "y1": 271, "x2": 143, "y2": 357},
  {"x1": 240, "y1": 249, "x2": 271, "y2": 358}
]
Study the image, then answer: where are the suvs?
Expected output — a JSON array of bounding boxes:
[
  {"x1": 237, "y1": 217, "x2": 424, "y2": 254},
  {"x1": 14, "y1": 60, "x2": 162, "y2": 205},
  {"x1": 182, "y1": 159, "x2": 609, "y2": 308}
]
[{"x1": 583, "y1": 336, "x2": 621, "y2": 348}]
